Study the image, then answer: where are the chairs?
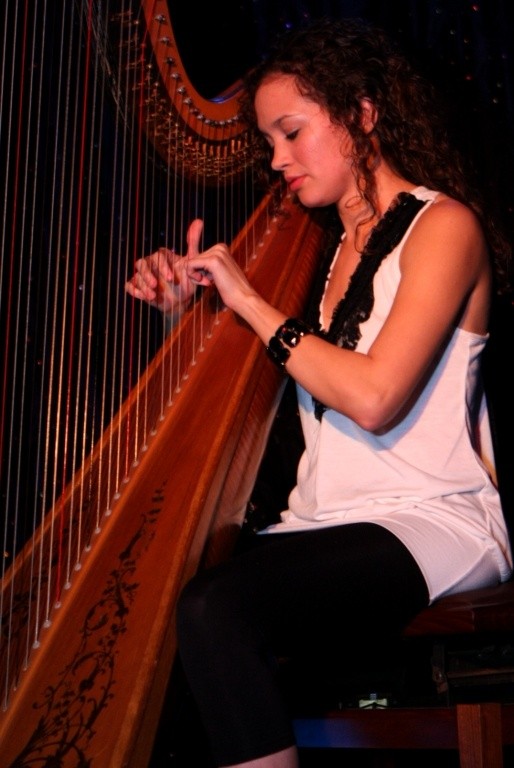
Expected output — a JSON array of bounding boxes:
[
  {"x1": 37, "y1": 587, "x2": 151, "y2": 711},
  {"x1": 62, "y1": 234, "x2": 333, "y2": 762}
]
[{"x1": 342, "y1": 576, "x2": 514, "y2": 768}]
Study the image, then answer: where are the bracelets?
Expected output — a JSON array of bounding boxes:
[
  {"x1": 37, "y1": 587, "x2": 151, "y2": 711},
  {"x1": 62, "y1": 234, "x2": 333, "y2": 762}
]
[{"x1": 264, "y1": 317, "x2": 312, "y2": 371}]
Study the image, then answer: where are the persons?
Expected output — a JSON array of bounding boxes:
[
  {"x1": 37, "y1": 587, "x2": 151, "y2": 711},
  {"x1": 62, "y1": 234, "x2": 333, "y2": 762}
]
[{"x1": 123, "y1": 20, "x2": 513, "y2": 768}]
